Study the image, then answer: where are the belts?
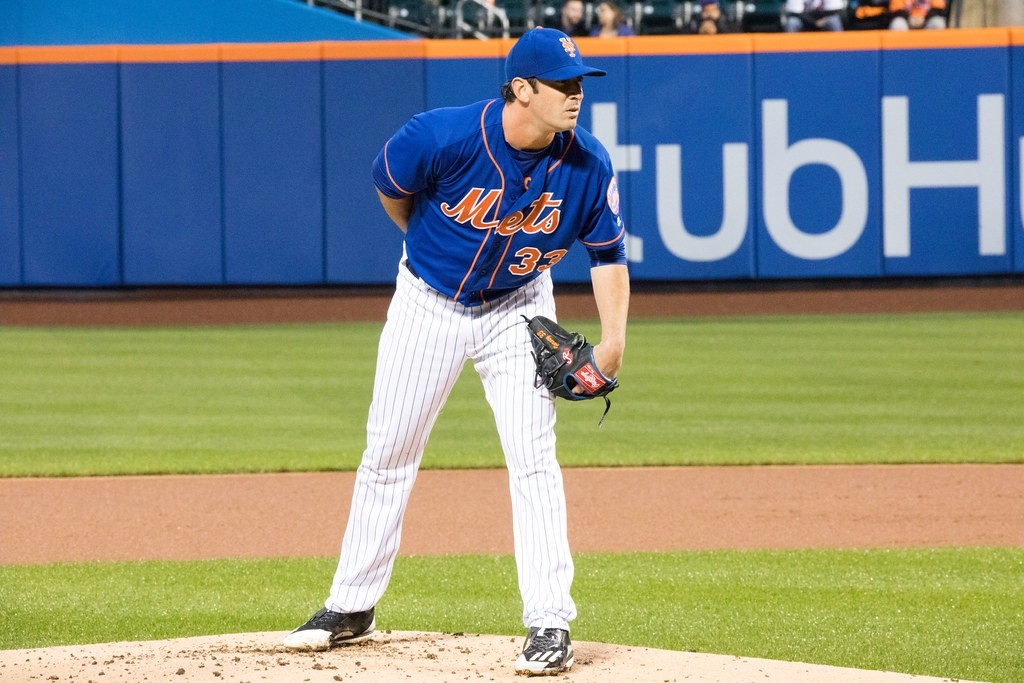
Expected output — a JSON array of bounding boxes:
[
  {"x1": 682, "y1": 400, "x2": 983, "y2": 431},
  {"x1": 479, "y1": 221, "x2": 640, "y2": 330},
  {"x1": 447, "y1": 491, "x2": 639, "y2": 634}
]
[{"x1": 404, "y1": 259, "x2": 419, "y2": 278}]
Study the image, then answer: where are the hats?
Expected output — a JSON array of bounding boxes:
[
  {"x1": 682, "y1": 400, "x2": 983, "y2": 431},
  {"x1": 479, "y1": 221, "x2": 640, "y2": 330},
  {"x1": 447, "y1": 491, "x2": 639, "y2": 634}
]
[{"x1": 504, "y1": 26, "x2": 608, "y2": 80}]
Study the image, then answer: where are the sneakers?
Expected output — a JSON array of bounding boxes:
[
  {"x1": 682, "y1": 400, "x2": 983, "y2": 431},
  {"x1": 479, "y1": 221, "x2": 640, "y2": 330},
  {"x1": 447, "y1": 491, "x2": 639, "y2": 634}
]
[
  {"x1": 283, "y1": 602, "x2": 375, "y2": 651},
  {"x1": 515, "y1": 628, "x2": 574, "y2": 674}
]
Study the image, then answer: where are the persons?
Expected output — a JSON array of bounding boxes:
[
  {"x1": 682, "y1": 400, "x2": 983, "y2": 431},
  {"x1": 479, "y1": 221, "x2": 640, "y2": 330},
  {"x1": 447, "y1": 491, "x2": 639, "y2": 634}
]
[
  {"x1": 589, "y1": 0, "x2": 636, "y2": 38},
  {"x1": 282, "y1": 25, "x2": 632, "y2": 675},
  {"x1": 686, "y1": 1, "x2": 743, "y2": 35},
  {"x1": 782, "y1": 0, "x2": 849, "y2": 32},
  {"x1": 887, "y1": 0, "x2": 947, "y2": 29},
  {"x1": 542, "y1": 0, "x2": 590, "y2": 39}
]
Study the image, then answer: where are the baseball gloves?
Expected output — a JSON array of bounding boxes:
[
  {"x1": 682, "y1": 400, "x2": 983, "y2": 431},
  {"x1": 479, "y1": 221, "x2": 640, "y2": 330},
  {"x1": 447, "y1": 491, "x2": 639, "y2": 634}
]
[{"x1": 525, "y1": 317, "x2": 620, "y2": 403}]
